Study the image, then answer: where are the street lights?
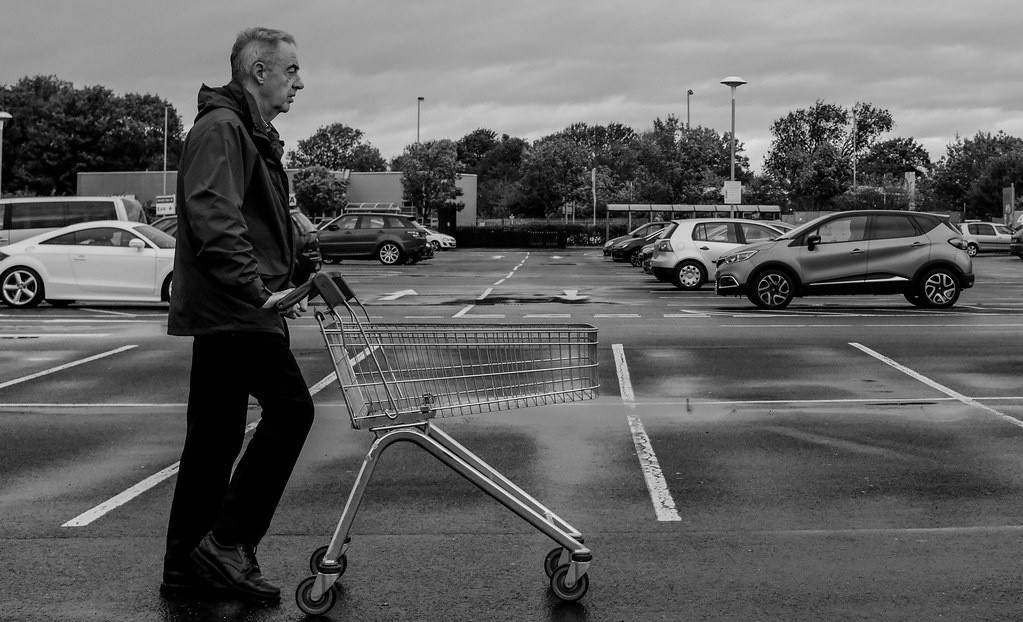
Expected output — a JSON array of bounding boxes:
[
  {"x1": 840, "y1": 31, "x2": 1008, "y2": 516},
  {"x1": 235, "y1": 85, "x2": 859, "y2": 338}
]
[
  {"x1": 686, "y1": 89, "x2": 694, "y2": 131},
  {"x1": 417, "y1": 96, "x2": 424, "y2": 143},
  {"x1": 852, "y1": 108, "x2": 859, "y2": 190},
  {"x1": 719, "y1": 76, "x2": 748, "y2": 221}
]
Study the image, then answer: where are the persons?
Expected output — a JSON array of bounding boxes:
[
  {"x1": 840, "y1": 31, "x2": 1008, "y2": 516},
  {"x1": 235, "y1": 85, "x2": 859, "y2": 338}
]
[{"x1": 159, "y1": 25, "x2": 307, "y2": 603}]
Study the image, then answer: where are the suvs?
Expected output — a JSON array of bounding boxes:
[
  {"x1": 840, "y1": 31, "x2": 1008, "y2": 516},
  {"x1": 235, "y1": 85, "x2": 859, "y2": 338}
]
[
  {"x1": 318, "y1": 212, "x2": 428, "y2": 265},
  {"x1": 954, "y1": 219, "x2": 1018, "y2": 258},
  {"x1": 149, "y1": 208, "x2": 323, "y2": 285},
  {"x1": 714, "y1": 209, "x2": 974, "y2": 312}
]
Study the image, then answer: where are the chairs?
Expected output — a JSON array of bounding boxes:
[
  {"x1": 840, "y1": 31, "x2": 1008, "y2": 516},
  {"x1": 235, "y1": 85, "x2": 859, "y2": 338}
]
[
  {"x1": 89, "y1": 231, "x2": 114, "y2": 245},
  {"x1": 848, "y1": 219, "x2": 863, "y2": 241}
]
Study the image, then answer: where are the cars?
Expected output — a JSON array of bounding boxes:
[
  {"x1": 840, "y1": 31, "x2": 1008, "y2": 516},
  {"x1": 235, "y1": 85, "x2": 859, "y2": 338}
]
[
  {"x1": 602, "y1": 222, "x2": 675, "y2": 256},
  {"x1": 346, "y1": 219, "x2": 435, "y2": 260},
  {"x1": 1010, "y1": 228, "x2": 1023, "y2": 259},
  {"x1": 649, "y1": 218, "x2": 784, "y2": 291},
  {"x1": 410, "y1": 220, "x2": 458, "y2": 251},
  {"x1": 611, "y1": 224, "x2": 722, "y2": 267},
  {"x1": 0, "y1": 220, "x2": 176, "y2": 310},
  {"x1": 637, "y1": 221, "x2": 836, "y2": 278}
]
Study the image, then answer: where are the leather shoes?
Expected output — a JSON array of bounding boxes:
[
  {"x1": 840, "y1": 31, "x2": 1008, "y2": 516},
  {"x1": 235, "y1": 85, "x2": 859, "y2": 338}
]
[
  {"x1": 190, "y1": 531, "x2": 280, "y2": 600},
  {"x1": 158, "y1": 567, "x2": 192, "y2": 599}
]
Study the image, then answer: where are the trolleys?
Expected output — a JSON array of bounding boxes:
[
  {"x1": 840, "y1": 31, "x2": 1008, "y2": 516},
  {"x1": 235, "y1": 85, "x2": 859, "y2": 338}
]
[{"x1": 273, "y1": 269, "x2": 601, "y2": 617}]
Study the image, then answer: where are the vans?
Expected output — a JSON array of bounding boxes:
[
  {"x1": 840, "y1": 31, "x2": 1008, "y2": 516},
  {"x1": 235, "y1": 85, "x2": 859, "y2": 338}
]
[{"x1": 0, "y1": 195, "x2": 148, "y2": 247}]
